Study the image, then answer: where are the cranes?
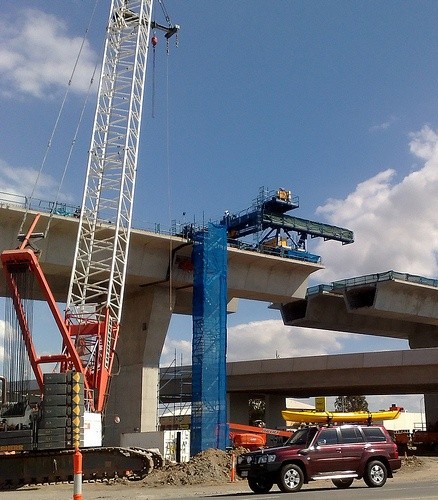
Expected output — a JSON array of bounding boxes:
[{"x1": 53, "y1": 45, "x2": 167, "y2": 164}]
[{"x1": 0, "y1": 0, "x2": 179, "y2": 491}]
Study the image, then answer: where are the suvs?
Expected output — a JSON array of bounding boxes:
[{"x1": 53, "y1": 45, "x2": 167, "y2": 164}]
[{"x1": 236, "y1": 423, "x2": 405, "y2": 492}]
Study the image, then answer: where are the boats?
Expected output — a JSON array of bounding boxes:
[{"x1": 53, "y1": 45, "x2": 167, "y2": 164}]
[{"x1": 281, "y1": 403, "x2": 405, "y2": 423}]
